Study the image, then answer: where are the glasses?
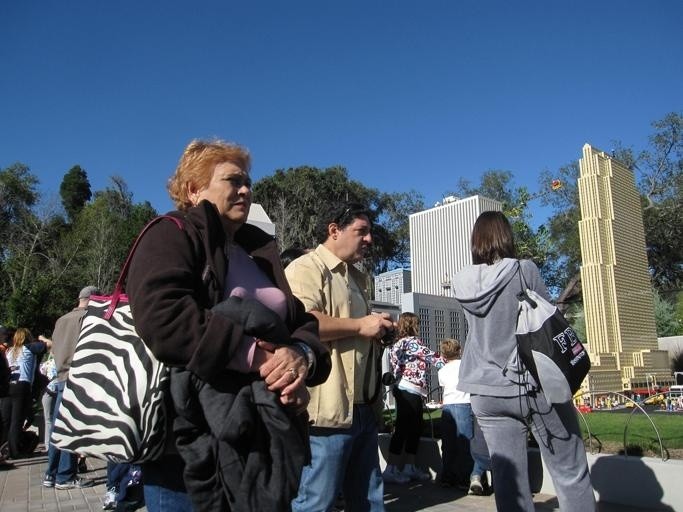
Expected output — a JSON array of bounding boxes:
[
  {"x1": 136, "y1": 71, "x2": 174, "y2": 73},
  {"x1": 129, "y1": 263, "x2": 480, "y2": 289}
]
[{"x1": 335, "y1": 203, "x2": 373, "y2": 223}]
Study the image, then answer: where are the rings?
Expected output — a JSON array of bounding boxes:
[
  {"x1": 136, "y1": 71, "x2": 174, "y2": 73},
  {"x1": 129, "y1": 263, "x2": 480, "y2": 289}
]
[{"x1": 288, "y1": 368, "x2": 302, "y2": 380}]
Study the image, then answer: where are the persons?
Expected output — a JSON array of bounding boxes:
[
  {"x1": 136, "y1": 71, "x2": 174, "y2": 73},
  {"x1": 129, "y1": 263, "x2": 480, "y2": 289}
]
[
  {"x1": 436, "y1": 338, "x2": 491, "y2": 494},
  {"x1": 1, "y1": 283, "x2": 102, "y2": 490},
  {"x1": 446, "y1": 206, "x2": 597, "y2": 511},
  {"x1": 385, "y1": 311, "x2": 444, "y2": 484},
  {"x1": 102, "y1": 462, "x2": 121, "y2": 511},
  {"x1": 283, "y1": 196, "x2": 386, "y2": 512},
  {"x1": 128, "y1": 138, "x2": 330, "y2": 510}
]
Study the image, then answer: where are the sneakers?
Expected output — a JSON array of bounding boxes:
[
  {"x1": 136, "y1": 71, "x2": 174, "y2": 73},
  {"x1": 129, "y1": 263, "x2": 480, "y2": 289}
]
[
  {"x1": 467, "y1": 476, "x2": 482, "y2": 495},
  {"x1": 402, "y1": 463, "x2": 430, "y2": 481},
  {"x1": 381, "y1": 464, "x2": 411, "y2": 484},
  {"x1": 44, "y1": 475, "x2": 93, "y2": 489},
  {"x1": 103, "y1": 491, "x2": 118, "y2": 510}
]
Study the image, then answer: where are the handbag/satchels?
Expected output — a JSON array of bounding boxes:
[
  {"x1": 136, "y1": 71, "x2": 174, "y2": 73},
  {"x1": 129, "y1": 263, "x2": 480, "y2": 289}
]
[
  {"x1": 513, "y1": 289, "x2": 589, "y2": 405},
  {"x1": 49, "y1": 294, "x2": 168, "y2": 464},
  {"x1": 381, "y1": 372, "x2": 394, "y2": 385}
]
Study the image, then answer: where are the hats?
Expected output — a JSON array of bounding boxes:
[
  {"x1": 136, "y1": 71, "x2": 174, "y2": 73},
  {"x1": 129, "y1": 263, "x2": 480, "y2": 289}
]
[{"x1": 79, "y1": 286, "x2": 104, "y2": 298}]
